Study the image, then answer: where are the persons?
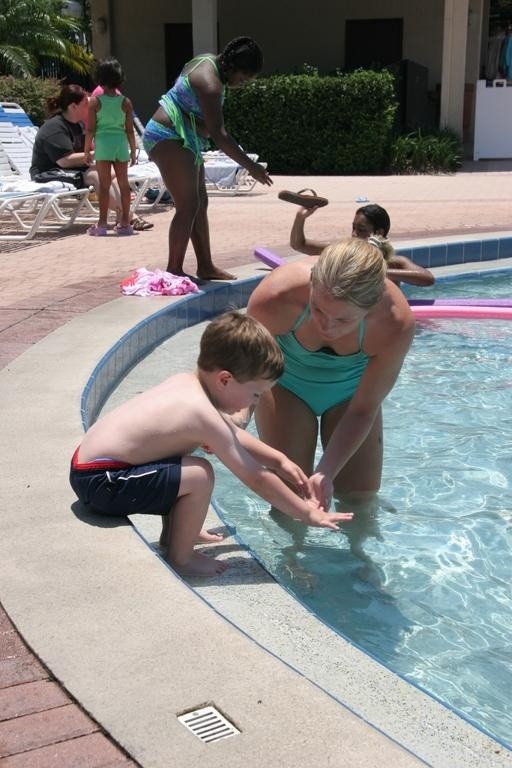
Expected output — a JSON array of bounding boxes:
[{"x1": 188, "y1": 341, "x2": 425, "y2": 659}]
[
  {"x1": 289, "y1": 203, "x2": 435, "y2": 300},
  {"x1": 200, "y1": 229, "x2": 418, "y2": 588},
  {"x1": 31, "y1": 84, "x2": 156, "y2": 230},
  {"x1": 86, "y1": 83, "x2": 123, "y2": 150},
  {"x1": 67, "y1": 299, "x2": 354, "y2": 576},
  {"x1": 83, "y1": 57, "x2": 139, "y2": 236},
  {"x1": 141, "y1": 35, "x2": 275, "y2": 286}
]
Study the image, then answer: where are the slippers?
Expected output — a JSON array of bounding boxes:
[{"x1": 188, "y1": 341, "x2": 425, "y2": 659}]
[
  {"x1": 277, "y1": 188, "x2": 327, "y2": 207},
  {"x1": 129, "y1": 217, "x2": 155, "y2": 230},
  {"x1": 356, "y1": 195, "x2": 371, "y2": 204}
]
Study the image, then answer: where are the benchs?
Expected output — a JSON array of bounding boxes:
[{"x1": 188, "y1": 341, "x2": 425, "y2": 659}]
[{"x1": 199, "y1": 145, "x2": 270, "y2": 197}]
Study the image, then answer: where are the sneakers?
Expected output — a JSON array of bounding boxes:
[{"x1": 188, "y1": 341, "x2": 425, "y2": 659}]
[
  {"x1": 84, "y1": 223, "x2": 105, "y2": 237},
  {"x1": 115, "y1": 224, "x2": 132, "y2": 234}
]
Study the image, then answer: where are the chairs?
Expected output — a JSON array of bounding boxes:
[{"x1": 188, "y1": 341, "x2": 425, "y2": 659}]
[{"x1": 0, "y1": 100, "x2": 168, "y2": 242}]
